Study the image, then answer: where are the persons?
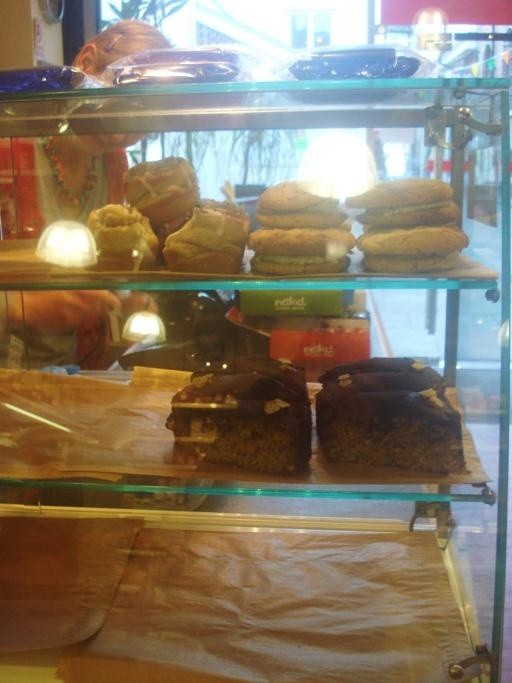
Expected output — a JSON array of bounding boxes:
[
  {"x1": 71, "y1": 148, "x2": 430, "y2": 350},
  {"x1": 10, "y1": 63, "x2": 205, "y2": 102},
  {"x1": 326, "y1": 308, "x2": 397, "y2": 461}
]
[{"x1": 1, "y1": 19, "x2": 176, "y2": 368}]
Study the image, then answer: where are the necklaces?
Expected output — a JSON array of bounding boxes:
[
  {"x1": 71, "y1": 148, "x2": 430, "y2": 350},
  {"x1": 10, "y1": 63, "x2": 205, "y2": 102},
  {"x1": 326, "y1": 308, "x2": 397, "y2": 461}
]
[{"x1": 38, "y1": 132, "x2": 99, "y2": 208}]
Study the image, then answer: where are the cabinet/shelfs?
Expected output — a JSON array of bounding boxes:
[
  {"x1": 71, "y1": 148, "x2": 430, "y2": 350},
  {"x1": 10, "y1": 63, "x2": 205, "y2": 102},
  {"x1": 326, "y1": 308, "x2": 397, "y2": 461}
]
[{"x1": 0, "y1": 77, "x2": 512, "y2": 683}]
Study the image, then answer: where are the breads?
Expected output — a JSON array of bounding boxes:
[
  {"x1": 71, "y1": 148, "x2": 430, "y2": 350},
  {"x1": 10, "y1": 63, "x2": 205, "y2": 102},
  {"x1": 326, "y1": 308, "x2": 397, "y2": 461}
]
[
  {"x1": 165, "y1": 354, "x2": 314, "y2": 477},
  {"x1": 91, "y1": 154, "x2": 251, "y2": 272},
  {"x1": 349, "y1": 175, "x2": 472, "y2": 272},
  {"x1": 256, "y1": 185, "x2": 358, "y2": 271},
  {"x1": 313, "y1": 355, "x2": 468, "y2": 478}
]
